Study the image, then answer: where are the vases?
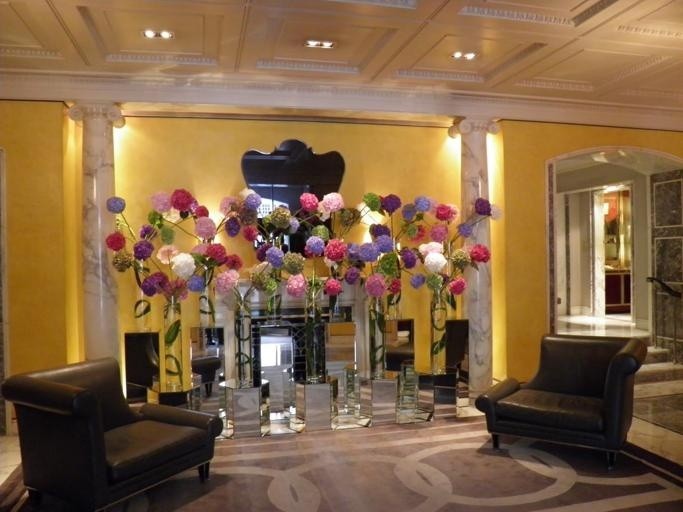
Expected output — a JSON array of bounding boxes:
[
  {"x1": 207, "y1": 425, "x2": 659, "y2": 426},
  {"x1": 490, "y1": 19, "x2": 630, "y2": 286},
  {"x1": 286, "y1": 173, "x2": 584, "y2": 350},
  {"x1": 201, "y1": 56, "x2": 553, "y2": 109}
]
[{"x1": 162, "y1": 287, "x2": 453, "y2": 386}]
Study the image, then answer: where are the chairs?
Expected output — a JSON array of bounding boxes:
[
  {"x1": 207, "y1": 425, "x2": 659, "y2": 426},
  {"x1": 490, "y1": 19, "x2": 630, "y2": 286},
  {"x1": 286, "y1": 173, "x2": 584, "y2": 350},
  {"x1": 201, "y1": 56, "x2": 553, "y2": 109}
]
[
  {"x1": 0, "y1": 355, "x2": 223, "y2": 511},
  {"x1": 474, "y1": 332, "x2": 648, "y2": 471}
]
